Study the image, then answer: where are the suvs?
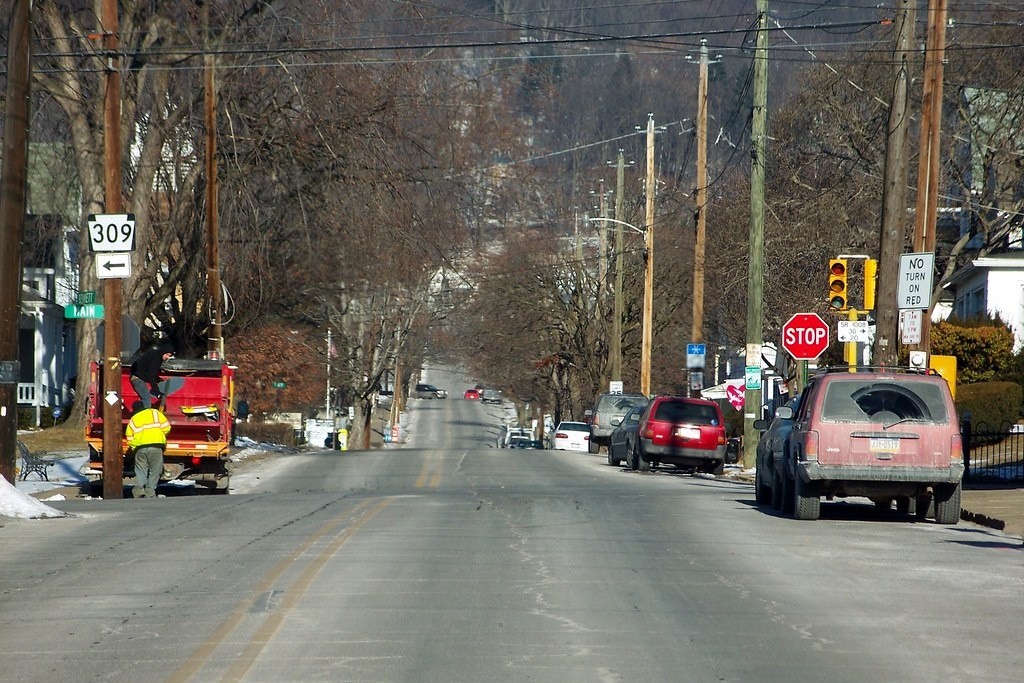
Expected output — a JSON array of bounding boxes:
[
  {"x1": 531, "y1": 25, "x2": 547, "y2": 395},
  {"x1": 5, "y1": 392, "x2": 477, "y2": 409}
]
[
  {"x1": 502, "y1": 430, "x2": 534, "y2": 447},
  {"x1": 584, "y1": 395, "x2": 649, "y2": 455},
  {"x1": 410, "y1": 384, "x2": 447, "y2": 400},
  {"x1": 772, "y1": 367, "x2": 966, "y2": 526},
  {"x1": 630, "y1": 396, "x2": 728, "y2": 477}
]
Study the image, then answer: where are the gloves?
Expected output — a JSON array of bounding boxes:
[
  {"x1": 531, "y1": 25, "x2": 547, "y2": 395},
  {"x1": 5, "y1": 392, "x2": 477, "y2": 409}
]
[{"x1": 157, "y1": 377, "x2": 164, "y2": 383}]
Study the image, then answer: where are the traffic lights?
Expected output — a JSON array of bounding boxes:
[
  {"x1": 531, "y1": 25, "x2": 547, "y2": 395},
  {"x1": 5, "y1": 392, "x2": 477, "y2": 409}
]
[{"x1": 829, "y1": 260, "x2": 848, "y2": 312}]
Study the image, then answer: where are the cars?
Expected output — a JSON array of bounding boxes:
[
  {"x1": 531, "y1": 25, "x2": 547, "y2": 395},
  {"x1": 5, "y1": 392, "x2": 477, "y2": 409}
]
[
  {"x1": 752, "y1": 395, "x2": 801, "y2": 517},
  {"x1": 481, "y1": 389, "x2": 501, "y2": 404},
  {"x1": 548, "y1": 421, "x2": 589, "y2": 453},
  {"x1": 474, "y1": 385, "x2": 487, "y2": 394},
  {"x1": 463, "y1": 390, "x2": 480, "y2": 399},
  {"x1": 606, "y1": 407, "x2": 646, "y2": 468},
  {"x1": 502, "y1": 437, "x2": 546, "y2": 451}
]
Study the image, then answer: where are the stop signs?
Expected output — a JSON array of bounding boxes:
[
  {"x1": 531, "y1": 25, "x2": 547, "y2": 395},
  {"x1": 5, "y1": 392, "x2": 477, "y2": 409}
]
[{"x1": 782, "y1": 313, "x2": 830, "y2": 360}]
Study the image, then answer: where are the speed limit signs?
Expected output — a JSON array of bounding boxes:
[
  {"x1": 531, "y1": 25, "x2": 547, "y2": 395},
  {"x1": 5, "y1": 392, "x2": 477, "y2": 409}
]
[{"x1": 609, "y1": 381, "x2": 623, "y2": 395}]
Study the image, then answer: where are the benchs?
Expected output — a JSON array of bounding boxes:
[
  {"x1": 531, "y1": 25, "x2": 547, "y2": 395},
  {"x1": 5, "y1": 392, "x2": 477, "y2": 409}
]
[{"x1": 16, "y1": 441, "x2": 54, "y2": 482}]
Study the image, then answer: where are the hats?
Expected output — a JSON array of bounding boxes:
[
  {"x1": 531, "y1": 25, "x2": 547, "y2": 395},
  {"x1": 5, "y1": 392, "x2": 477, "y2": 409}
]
[{"x1": 132, "y1": 401, "x2": 144, "y2": 411}]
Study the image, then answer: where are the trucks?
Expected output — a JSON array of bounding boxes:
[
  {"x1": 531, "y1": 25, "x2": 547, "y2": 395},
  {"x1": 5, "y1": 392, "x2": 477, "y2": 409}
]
[{"x1": 82, "y1": 358, "x2": 236, "y2": 494}]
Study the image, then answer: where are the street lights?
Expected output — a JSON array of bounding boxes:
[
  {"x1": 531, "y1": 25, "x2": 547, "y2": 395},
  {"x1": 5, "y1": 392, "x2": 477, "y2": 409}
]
[
  {"x1": 292, "y1": 329, "x2": 330, "y2": 419},
  {"x1": 579, "y1": 209, "x2": 651, "y2": 393}
]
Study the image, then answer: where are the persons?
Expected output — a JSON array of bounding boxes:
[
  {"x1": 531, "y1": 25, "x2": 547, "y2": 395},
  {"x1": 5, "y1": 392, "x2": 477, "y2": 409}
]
[
  {"x1": 125, "y1": 408, "x2": 171, "y2": 498},
  {"x1": 130, "y1": 345, "x2": 174, "y2": 409}
]
